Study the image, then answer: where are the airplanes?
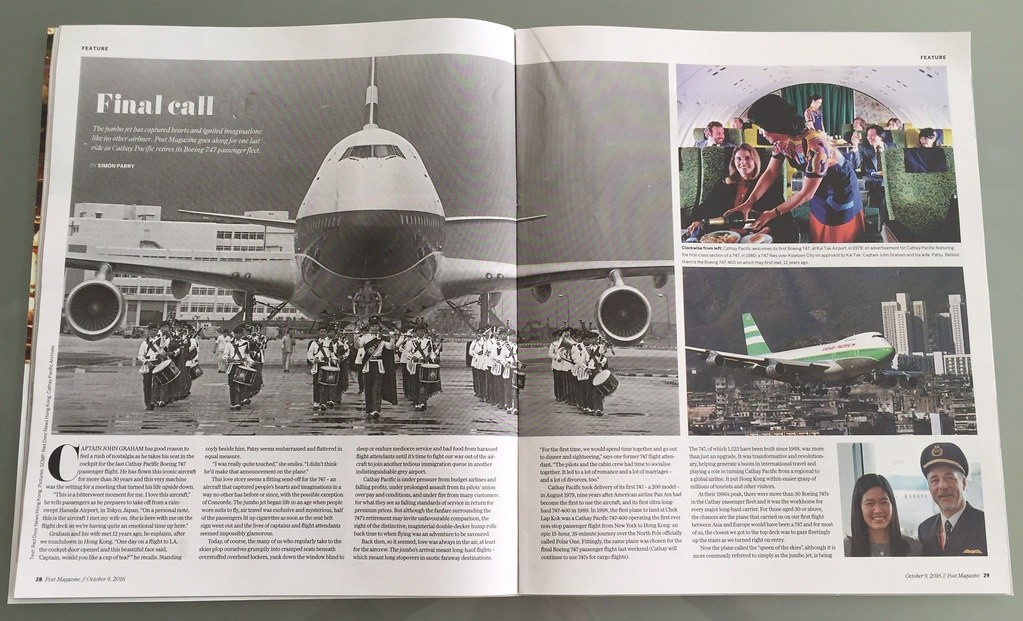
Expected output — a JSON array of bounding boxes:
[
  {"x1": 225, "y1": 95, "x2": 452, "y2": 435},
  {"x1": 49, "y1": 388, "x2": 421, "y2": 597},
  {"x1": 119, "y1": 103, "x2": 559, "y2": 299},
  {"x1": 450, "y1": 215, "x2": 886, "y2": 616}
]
[{"x1": 64, "y1": 57, "x2": 674, "y2": 347}]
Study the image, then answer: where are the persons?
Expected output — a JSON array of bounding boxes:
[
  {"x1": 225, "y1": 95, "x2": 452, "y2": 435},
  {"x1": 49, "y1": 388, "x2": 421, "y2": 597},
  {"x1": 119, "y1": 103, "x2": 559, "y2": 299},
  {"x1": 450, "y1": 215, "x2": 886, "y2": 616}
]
[
  {"x1": 307, "y1": 316, "x2": 442, "y2": 417},
  {"x1": 548, "y1": 328, "x2": 608, "y2": 415},
  {"x1": 844, "y1": 443, "x2": 988, "y2": 558},
  {"x1": 470, "y1": 325, "x2": 526, "y2": 413},
  {"x1": 213, "y1": 326, "x2": 267, "y2": 409},
  {"x1": 281, "y1": 329, "x2": 296, "y2": 372},
  {"x1": 686, "y1": 95, "x2": 938, "y2": 243},
  {"x1": 138, "y1": 320, "x2": 200, "y2": 410}
]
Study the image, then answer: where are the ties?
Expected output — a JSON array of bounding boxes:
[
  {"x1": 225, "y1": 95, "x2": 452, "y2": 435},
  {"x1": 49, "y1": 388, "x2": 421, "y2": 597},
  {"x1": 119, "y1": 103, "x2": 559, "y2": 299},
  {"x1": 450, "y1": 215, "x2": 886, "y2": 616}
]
[
  {"x1": 943, "y1": 520, "x2": 952, "y2": 552},
  {"x1": 712, "y1": 144, "x2": 717, "y2": 147},
  {"x1": 876, "y1": 145, "x2": 882, "y2": 172}
]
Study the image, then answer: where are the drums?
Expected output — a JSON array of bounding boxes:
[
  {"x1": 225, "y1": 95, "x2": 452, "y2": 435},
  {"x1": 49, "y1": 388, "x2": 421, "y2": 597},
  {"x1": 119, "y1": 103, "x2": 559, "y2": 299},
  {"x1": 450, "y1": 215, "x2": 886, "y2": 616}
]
[
  {"x1": 418, "y1": 363, "x2": 441, "y2": 384},
  {"x1": 189, "y1": 362, "x2": 204, "y2": 380},
  {"x1": 511, "y1": 370, "x2": 526, "y2": 389},
  {"x1": 592, "y1": 370, "x2": 621, "y2": 397},
  {"x1": 151, "y1": 358, "x2": 181, "y2": 385},
  {"x1": 318, "y1": 365, "x2": 342, "y2": 387},
  {"x1": 233, "y1": 365, "x2": 259, "y2": 386}
]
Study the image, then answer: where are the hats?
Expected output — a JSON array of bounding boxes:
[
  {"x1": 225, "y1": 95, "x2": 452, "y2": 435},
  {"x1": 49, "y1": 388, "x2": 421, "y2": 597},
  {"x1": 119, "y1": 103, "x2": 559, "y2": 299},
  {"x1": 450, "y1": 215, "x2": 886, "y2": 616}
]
[
  {"x1": 920, "y1": 442, "x2": 969, "y2": 477},
  {"x1": 553, "y1": 329, "x2": 600, "y2": 339},
  {"x1": 477, "y1": 324, "x2": 515, "y2": 335},
  {"x1": 146, "y1": 317, "x2": 195, "y2": 332},
  {"x1": 317, "y1": 316, "x2": 428, "y2": 339},
  {"x1": 231, "y1": 322, "x2": 265, "y2": 339}
]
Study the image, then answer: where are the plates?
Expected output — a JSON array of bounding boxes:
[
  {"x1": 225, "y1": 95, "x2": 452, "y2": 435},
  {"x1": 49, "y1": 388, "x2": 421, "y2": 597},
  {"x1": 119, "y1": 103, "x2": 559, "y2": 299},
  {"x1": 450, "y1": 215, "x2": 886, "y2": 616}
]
[
  {"x1": 700, "y1": 230, "x2": 741, "y2": 243},
  {"x1": 741, "y1": 234, "x2": 772, "y2": 243}
]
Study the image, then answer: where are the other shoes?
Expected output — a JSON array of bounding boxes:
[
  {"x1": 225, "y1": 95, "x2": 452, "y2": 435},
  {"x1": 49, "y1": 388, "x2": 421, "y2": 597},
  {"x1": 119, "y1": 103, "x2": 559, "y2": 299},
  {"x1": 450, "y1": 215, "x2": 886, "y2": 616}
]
[{"x1": 136, "y1": 366, "x2": 605, "y2": 417}]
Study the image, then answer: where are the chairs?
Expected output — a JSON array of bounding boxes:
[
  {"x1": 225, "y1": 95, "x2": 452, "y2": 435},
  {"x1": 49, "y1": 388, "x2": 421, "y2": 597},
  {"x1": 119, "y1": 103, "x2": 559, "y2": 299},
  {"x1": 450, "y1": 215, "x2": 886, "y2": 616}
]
[{"x1": 678, "y1": 124, "x2": 957, "y2": 244}]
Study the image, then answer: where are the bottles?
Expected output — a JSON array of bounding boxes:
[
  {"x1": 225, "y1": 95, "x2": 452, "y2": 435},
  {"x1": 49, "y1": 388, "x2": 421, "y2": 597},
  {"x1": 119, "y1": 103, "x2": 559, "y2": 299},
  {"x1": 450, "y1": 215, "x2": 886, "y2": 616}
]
[{"x1": 707, "y1": 212, "x2": 769, "y2": 229}]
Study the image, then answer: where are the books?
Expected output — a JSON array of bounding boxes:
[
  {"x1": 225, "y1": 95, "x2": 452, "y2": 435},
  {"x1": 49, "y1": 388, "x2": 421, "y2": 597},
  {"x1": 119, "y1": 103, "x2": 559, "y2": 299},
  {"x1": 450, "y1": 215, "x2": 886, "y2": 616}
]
[{"x1": 7, "y1": 18, "x2": 1014, "y2": 597}]
